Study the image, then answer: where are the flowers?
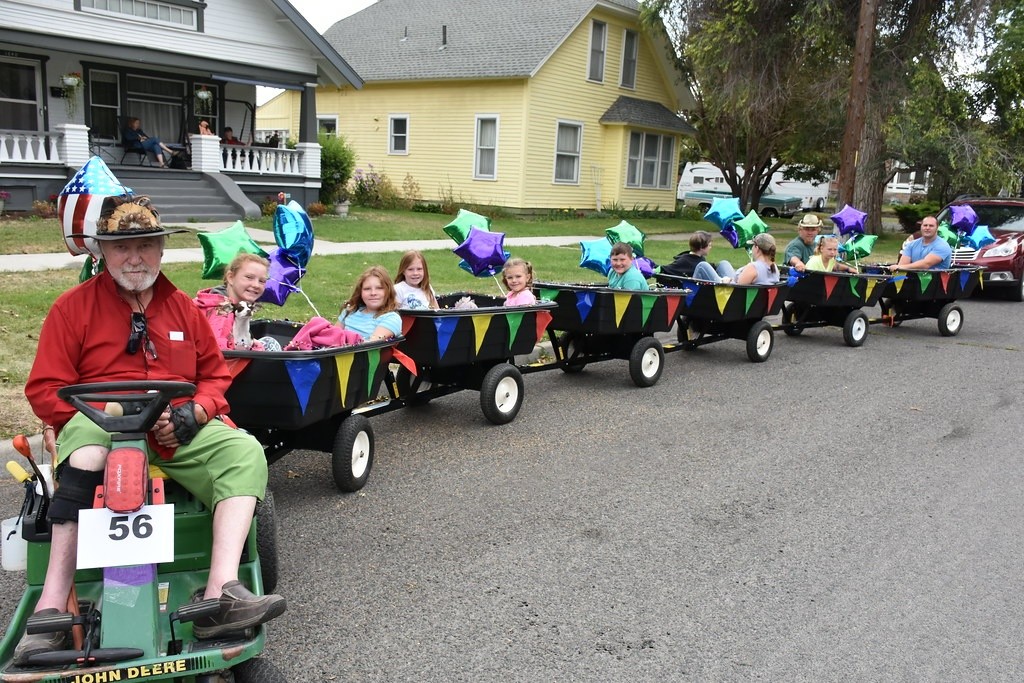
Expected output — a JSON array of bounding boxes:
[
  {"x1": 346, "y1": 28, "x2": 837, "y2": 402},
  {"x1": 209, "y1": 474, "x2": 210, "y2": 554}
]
[
  {"x1": 59, "y1": 72, "x2": 85, "y2": 120},
  {"x1": 193, "y1": 86, "x2": 213, "y2": 125},
  {"x1": 0, "y1": 191, "x2": 11, "y2": 201}
]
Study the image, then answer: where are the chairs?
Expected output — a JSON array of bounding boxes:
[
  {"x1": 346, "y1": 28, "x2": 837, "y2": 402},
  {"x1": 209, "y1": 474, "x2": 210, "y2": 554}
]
[{"x1": 115, "y1": 116, "x2": 146, "y2": 166}]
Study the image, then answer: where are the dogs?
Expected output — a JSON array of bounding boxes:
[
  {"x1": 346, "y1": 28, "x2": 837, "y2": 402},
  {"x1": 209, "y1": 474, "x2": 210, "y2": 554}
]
[{"x1": 230, "y1": 300, "x2": 262, "y2": 351}]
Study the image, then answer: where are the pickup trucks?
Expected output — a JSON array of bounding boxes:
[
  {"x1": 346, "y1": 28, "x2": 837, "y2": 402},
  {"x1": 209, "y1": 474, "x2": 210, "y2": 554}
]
[{"x1": 684, "y1": 183, "x2": 804, "y2": 219}]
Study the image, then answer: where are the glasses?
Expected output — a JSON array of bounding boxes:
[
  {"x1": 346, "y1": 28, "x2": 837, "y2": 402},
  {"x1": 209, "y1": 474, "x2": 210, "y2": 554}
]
[
  {"x1": 126, "y1": 312, "x2": 147, "y2": 355},
  {"x1": 752, "y1": 235, "x2": 757, "y2": 245}
]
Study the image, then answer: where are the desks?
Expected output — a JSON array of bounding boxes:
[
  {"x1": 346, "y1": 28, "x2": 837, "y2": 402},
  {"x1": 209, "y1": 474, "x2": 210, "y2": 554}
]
[{"x1": 160, "y1": 146, "x2": 188, "y2": 168}]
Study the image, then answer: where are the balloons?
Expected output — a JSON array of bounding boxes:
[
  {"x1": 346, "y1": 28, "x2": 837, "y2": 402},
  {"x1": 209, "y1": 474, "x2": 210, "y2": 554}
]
[
  {"x1": 580, "y1": 237, "x2": 613, "y2": 277},
  {"x1": 937, "y1": 204, "x2": 996, "y2": 251},
  {"x1": 58, "y1": 157, "x2": 132, "y2": 261},
  {"x1": 830, "y1": 204, "x2": 868, "y2": 236},
  {"x1": 443, "y1": 209, "x2": 492, "y2": 246},
  {"x1": 843, "y1": 234, "x2": 878, "y2": 261},
  {"x1": 453, "y1": 224, "x2": 511, "y2": 279},
  {"x1": 605, "y1": 220, "x2": 646, "y2": 254},
  {"x1": 703, "y1": 197, "x2": 769, "y2": 248},
  {"x1": 273, "y1": 200, "x2": 315, "y2": 267},
  {"x1": 197, "y1": 220, "x2": 269, "y2": 282},
  {"x1": 257, "y1": 247, "x2": 306, "y2": 307},
  {"x1": 631, "y1": 253, "x2": 655, "y2": 280}
]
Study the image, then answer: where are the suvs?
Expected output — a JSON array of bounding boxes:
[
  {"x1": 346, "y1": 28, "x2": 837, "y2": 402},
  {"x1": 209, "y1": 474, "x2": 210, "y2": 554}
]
[{"x1": 897, "y1": 192, "x2": 1024, "y2": 303}]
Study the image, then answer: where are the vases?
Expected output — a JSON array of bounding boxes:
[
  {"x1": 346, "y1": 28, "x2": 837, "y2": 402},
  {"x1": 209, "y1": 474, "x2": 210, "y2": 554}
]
[
  {"x1": 63, "y1": 78, "x2": 79, "y2": 86},
  {"x1": 0, "y1": 200, "x2": 5, "y2": 215},
  {"x1": 333, "y1": 203, "x2": 351, "y2": 216},
  {"x1": 197, "y1": 91, "x2": 212, "y2": 100}
]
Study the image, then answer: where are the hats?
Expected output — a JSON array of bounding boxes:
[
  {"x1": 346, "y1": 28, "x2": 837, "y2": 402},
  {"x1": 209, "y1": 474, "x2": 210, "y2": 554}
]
[
  {"x1": 799, "y1": 214, "x2": 822, "y2": 228},
  {"x1": 747, "y1": 233, "x2": 776, "y2": 250},
  {"x1": 65, "y1": 201, "x2": 192, "y2": 242}
]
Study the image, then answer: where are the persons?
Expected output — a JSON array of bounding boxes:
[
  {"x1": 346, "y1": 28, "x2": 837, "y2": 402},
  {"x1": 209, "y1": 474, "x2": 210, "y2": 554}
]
[
  {"x1": 693, "y1": 233, "x2": 780, "y2": 286},
  {"x1": 126, "y1": 118, "x2": 178, "y2": 168},
  {"x1": 192, "y1": 254, "x2": 282, "y2": 352},
  {"x1": 784, "y1": 214, "x2": 822, "y2": 273},
  {"x1": 806, "y1": 236, "x2": 858, "y2": 274},
  {"x1": 607, "y1": 242, "x2": 649, "y2": 291},
  {"x1": 222, "y1": 127, "x2": 246, "y2": 145},
  {"x1": 334, "y1": 265, "x2": 403, "y2": 342},
  {"x1": 393, "y1": 251, "x2": 440, "y2": 309},
  {"x1": 890, "y1": 215, "x2": 952, "y2": 273},
  {"x1": 12, "y1": 203, "x2": 286, "y2": 669},
  {"x1": 502, "y1": 258, "x2": 536, "y2": 306},
  {"x1": 661, "y1": 231, "x2": 713, "y2": 276}
]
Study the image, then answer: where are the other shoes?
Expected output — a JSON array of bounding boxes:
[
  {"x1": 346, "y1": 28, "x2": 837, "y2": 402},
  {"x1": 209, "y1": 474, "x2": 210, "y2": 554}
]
[
  {"x1": 162, "y1": 164, "x2": 169, "y2": 169},
  {"x1": 13, "y1": 608, "x2": 69, "y2": 666},
  {"x1": 171, "y1": 150, "x2": 180, "y2": 156},
  {"x1": 192, "y1": 580, "x2": 288, "y2": 639}
]
